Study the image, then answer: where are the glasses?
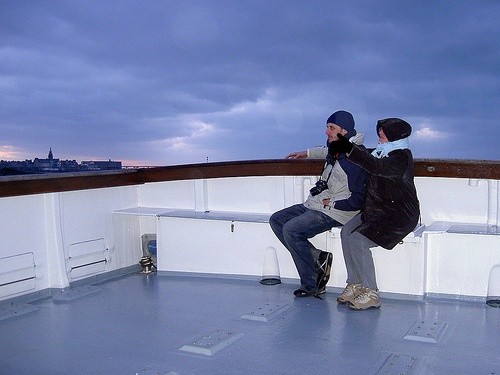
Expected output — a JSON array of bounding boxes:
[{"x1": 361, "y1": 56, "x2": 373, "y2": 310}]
[{"x1": 378, "y1": 128, "x2": 383, "y2": 131}]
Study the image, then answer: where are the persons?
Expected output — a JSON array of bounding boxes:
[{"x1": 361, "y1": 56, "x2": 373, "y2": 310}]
[
  {"x1": 269, "y1": 110, "x2": 369, "y2": 300},
  {"x1": 331, "y1": 118, "x2": 420, "y2": 310}
]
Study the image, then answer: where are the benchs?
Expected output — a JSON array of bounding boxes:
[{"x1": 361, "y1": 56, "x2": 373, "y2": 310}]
[{"x1": 114, "y1": 207, "x2": 500, "y2": 303}]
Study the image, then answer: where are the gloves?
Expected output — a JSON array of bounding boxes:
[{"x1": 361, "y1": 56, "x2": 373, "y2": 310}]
[{"x1": 330, "y1": 133, "x2": 353, "y2": 154}]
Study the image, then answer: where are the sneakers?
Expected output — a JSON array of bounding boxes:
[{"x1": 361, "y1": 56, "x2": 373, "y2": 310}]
[
  {"x1": 315, "y1": 251, "x2": 333, "y2": 293},
  {"x1": 336, "y1": 283, "x2": 381, "y2": 309},
  {"x1": 293, "y1": 287, "x2": 326, "y2": 297}
]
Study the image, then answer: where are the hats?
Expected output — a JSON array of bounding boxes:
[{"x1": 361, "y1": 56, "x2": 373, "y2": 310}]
[{"x1": 327, "y1": 111, "x2": 355, "y2": 132}]
